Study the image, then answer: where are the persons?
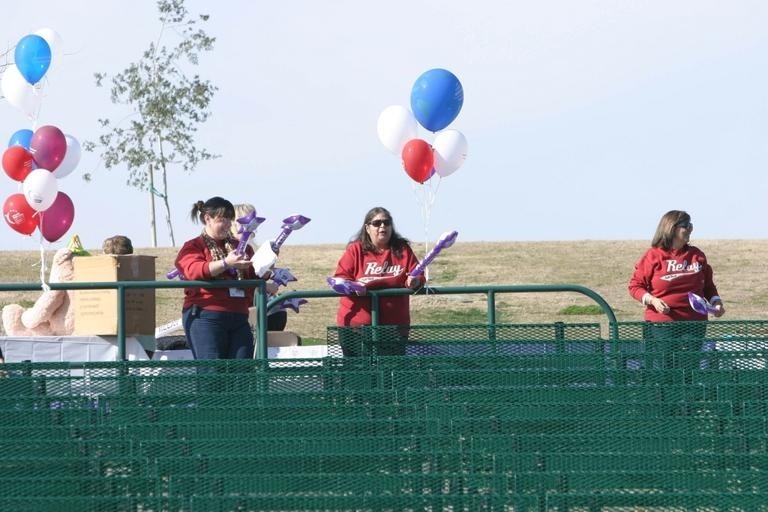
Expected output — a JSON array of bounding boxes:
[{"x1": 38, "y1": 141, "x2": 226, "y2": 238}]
[
  {"x1": 329, "y1": 208, "x2": 426, "y2": 355},
  {"x1": 105, "y1": 234, "x2": 132, "y2": 255},
  {"x1": 174, "y1": 196, "x2": 263, "y2": 360},
  {"x1": 630, "y1": 211, "x2": 726, "y2": 364}
]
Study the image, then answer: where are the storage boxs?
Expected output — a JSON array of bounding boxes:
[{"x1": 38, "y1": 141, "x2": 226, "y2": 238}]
[{"x1": 73, "y1": 255, "x2": 155, "y2": 336}]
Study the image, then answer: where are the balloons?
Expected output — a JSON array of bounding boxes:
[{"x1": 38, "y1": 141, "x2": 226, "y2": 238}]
[
  {"x1": 411, "y1": 68, "x2": 463, "y2": 131},
  {"x1": 38, "y1": 193, "x2": 74, "y2": 242},
  {"x1": 4, "y1": 194, "x2": 39, "y2": 236},
  {"x1": 15, "y1": 35, "x2": 51, "y2": 87},
  {"x1": 9, "y1": 130, "x2": 33, "y2": 152},
  {"x1": 54, "y1": 133, "x2": 83, "y2": 179},
  {"x1": 2, "y1": 146, "x2": 31, "y2": 183},
  {"x1": 30, "y1": 126, "x2": 66, "y2": 172},
  {"x1": 402, "y1": 140, "x2": 433, "y2": 183},
  {"x1": 430, "y1": 129, "x2": 468, "y2": 178},
  {"x1": 23, "y1": 168, "x2": 59, "y2": 210}
]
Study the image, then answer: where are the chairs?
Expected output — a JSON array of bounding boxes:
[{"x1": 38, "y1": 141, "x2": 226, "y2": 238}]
[
  {"x1": 157, "y1": 334, "x2": 191, "y2": 351},
  {"x1": 267, "y1": 331, "x2": 302, "y2": 347}
]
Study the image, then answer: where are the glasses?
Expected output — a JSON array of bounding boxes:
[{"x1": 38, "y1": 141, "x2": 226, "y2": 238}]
[
  {"x1": 674, "y1": 214, "x2": 693, "y2": 229},
  {"x1": 364, "y1": 218, "x2": 395, "y2": 230}
]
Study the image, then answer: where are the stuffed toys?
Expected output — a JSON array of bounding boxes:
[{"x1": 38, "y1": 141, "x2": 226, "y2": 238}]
[{"x1": 3, "y1": 247, "x2": 86, "y2": 336}]
